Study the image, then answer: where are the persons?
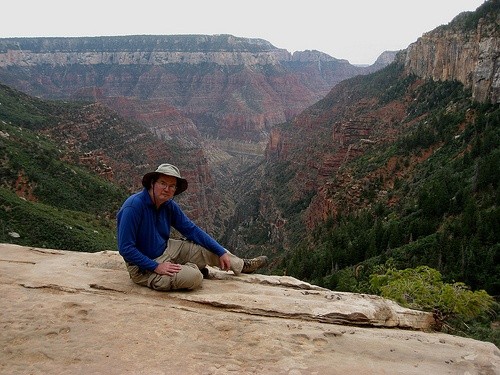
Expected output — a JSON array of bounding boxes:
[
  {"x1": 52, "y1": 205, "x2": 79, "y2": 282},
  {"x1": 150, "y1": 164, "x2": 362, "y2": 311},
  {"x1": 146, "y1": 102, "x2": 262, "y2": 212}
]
[{"x1": 115, "y1": 163, "x2": 269, "y2": 291}]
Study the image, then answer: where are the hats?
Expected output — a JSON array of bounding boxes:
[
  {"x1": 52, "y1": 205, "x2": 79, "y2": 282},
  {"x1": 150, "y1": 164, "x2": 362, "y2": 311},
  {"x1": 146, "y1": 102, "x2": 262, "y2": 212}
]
[{"x1": 142, "y1": 163, "x2": 188, "y2": 196}]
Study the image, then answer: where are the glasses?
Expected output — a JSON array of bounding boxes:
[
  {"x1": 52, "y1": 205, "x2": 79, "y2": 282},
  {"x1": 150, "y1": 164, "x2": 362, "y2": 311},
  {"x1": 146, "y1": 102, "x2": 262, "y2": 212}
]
[{"x1": 156, "y1": 181, "x2": 177, "y2": 192}]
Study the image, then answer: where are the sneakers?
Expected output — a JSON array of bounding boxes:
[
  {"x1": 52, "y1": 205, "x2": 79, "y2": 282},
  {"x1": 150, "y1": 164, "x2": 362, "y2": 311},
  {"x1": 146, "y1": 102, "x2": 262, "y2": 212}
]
[
  {"x1": 200, "y1": 268, "x2": 209, "y2": 279},
  {"x1": 241, "y1": 256, "x2": 269, "y2": 274}
]
